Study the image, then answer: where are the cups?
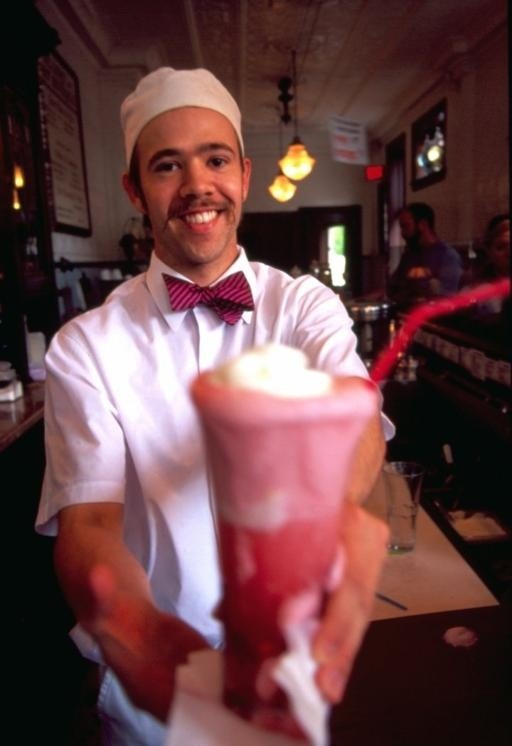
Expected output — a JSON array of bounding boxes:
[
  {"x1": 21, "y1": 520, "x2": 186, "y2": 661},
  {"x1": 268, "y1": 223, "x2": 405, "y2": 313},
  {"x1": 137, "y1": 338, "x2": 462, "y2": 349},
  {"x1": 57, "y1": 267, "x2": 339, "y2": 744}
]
[{"x1": 382, "y1": 461, "x2": 422, "y2": 555}]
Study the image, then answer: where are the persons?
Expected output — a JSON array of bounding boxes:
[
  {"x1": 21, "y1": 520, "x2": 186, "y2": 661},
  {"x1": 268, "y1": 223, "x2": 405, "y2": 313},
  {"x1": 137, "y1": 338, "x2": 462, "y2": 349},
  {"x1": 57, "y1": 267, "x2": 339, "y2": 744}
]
[
  {"x1": 32, "y1": 65, "x2": 398, "y2": 745},
  {"x1": 389, "y1": 201, "x2": 460, "y2": 324},
  {"x1": 479, "y1": 212, "x2": 511, "y2": 361}
]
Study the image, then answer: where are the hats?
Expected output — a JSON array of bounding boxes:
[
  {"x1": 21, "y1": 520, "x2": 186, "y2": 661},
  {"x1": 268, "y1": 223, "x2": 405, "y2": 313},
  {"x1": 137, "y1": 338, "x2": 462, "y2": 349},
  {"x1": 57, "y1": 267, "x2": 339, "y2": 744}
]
[{"x1": 119, "y1": 65, "x2": 245, "y2": 177}]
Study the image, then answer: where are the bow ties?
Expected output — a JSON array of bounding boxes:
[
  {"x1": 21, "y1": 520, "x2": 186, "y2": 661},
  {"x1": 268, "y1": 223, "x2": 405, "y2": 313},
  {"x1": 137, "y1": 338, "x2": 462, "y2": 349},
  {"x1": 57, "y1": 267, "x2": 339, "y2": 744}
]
[{"x1": 161, "y1": 270, "x2": 254, "y2": 326}]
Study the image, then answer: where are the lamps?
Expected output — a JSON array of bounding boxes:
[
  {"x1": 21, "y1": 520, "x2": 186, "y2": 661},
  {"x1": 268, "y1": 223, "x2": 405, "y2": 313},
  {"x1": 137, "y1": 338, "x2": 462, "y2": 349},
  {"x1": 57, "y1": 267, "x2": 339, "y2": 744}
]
[
  {"x1": 268, "y1": 108, "x2": 297, "y2": 203},
  {"x1": 279, "y1": 49, "x2": 315, "y2": 182}
]
